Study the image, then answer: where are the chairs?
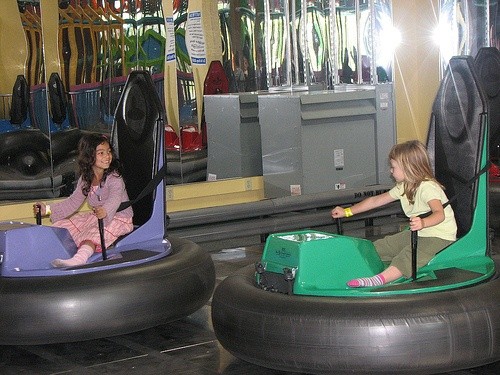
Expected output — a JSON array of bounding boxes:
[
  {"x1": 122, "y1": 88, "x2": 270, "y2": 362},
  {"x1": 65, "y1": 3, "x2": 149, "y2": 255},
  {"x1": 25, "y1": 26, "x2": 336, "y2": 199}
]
[
  {"x1": 474, "y1": 47, "x2": 500, "y2": 167},
  {"x1": 36, "y1": 70, "x2": 166, "y2": 260},
  {"x1": 9, "y1": 72, "x2": 67, "y2": 126},
  {"x1": 336, "y1": 55, "x2": 490, "y2": 278},
  {"x1": 201, "y1": 60, "x2": 229, "y2": 147}
]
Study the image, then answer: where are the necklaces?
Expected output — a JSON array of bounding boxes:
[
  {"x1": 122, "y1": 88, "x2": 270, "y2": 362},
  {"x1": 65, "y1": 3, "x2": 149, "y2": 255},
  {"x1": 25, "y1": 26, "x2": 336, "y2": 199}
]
[{"x1": 91, "y1": 186, "x2": 102, "y2": 201}]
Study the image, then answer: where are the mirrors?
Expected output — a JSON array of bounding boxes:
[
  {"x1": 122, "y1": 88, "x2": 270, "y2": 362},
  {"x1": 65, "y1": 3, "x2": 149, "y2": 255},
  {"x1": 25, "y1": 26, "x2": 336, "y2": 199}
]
[{"x1": 0, "y1": 0, "x2": 500, "y2": 226}]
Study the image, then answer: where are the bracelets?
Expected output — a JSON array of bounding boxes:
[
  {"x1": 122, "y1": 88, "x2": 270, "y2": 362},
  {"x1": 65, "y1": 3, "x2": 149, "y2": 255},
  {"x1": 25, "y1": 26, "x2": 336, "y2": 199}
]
[
  {"x1": 46, "y1": 205, "x2": 51, "y2": 215},
  {"x1": 345, "y1": 208, "x2": 353, "y2": 217}
]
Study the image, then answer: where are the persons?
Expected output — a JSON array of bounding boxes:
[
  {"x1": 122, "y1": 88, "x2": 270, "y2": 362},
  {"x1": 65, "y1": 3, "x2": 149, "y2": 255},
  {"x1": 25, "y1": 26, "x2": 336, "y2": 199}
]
[
  {"x1": 331, "y1": 141, "x2": 457, "y2": 287},
  {"x1": 32, "y1": 134, "x2": 135, "y2": 267}
]
